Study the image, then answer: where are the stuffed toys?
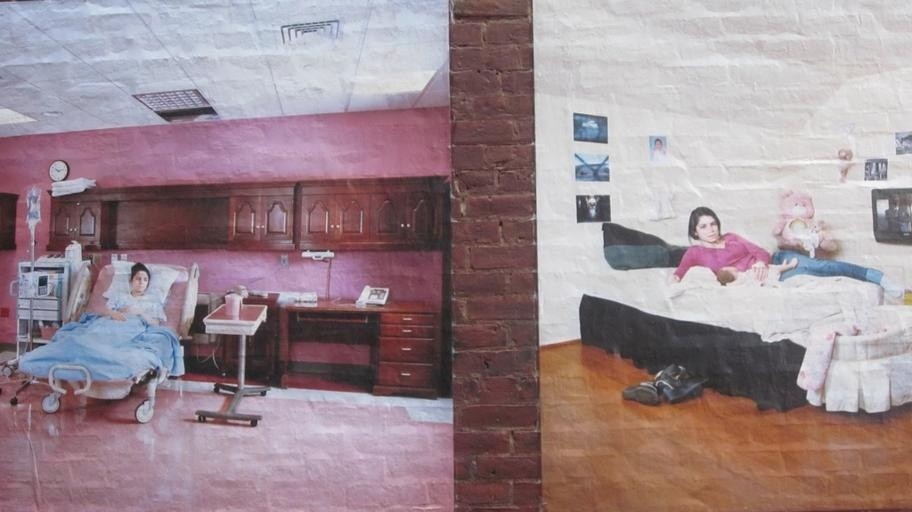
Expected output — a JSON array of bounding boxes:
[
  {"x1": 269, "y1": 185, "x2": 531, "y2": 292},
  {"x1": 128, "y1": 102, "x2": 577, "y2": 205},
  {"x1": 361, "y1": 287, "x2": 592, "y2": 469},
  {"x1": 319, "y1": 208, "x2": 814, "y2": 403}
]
[{"x1": 774, "y1": 187, "x2": 839, "y2": 256}]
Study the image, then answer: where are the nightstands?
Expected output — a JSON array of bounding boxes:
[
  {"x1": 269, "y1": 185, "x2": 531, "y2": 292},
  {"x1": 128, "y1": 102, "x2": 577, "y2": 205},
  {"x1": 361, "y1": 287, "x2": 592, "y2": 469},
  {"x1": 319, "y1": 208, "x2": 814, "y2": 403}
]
[{"x1": 227, "y1": 293, "x2": 279, "y2": 382}]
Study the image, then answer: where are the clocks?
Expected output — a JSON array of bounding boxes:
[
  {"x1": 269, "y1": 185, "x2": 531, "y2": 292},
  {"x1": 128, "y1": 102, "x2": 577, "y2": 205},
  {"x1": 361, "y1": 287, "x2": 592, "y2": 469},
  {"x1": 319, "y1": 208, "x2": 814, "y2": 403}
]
[{"x1": 49, "y1": 160, "x2": 69, "y2": 181}]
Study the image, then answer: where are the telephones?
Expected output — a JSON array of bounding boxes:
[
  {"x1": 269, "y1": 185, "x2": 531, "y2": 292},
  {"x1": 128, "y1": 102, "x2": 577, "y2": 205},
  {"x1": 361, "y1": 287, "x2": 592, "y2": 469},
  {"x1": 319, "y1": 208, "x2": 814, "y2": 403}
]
[{"x1": 355, "y1": 284, "x2": 390, "y2": 307}]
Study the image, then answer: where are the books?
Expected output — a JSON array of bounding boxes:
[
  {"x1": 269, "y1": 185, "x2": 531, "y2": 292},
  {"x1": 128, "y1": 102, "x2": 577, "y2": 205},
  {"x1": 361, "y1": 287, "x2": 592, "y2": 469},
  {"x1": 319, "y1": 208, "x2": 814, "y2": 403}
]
[{"x1": 358, "y1": 285, "x2": 389, "y2": 305}]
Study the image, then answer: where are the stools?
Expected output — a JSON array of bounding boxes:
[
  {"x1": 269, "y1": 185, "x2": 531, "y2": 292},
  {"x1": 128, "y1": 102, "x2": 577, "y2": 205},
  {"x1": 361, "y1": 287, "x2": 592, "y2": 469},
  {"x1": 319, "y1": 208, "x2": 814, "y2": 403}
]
[{"x1": 822, "y1": 352, "x2": 911, "y2": 414}]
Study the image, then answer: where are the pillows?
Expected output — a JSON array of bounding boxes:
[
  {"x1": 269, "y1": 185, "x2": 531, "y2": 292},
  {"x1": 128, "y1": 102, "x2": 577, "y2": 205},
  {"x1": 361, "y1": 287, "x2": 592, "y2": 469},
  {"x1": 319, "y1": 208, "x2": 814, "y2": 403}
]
[
  {"x1": 672, "y1": 243, "x2": 688, "y2": 267},
  {"x1": 103, "y1": 259, "x2": 177, "y2": 310},
  {"x1": 602, "y1": 223, "x2": 671, "y2": 271}
]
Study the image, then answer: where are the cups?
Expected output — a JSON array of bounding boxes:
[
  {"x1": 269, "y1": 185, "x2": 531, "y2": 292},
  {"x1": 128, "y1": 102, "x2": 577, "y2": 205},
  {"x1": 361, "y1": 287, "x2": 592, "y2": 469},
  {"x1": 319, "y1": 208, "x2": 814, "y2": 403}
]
[{"x1": 223, "y1": 293, "x2": 243, "y2": 316}]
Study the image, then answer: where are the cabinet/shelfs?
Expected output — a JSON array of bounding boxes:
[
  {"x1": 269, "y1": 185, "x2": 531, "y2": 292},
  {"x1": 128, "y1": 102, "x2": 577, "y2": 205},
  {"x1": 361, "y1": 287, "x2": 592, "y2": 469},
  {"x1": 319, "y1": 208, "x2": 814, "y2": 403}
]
[
  {"x1": 371, "y1": 176, "x2": 452, "y2": 251},
  {"x1": 10, "y1": 255, "x2": 93, "y2": 360},
  {"x1": 228, "y1": 181, "x2": 295, "y2": 251},
  {"x1": 46, "y1": 189, "x2": 103, "y2": 251},
  {"x1": 296, "y1": 179, "x2": 373, "y2": 252}
]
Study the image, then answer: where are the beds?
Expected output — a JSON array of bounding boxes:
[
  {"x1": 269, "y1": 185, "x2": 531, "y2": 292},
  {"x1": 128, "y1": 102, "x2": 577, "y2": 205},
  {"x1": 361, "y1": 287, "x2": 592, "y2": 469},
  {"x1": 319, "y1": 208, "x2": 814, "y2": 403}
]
[
  {"x1": 10, "y1": 261, "x2": 199, "y2": 423},
  {"x1": 578, "y1": 223, "x2": 906, "y2": 412}
]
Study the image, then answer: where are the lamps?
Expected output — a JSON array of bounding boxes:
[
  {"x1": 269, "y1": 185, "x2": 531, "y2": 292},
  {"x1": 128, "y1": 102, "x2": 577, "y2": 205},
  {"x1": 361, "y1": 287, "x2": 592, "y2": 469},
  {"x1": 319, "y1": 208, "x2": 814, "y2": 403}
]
[{"x1": 301, "y1": 249, "x2": 336, "y2": 301}]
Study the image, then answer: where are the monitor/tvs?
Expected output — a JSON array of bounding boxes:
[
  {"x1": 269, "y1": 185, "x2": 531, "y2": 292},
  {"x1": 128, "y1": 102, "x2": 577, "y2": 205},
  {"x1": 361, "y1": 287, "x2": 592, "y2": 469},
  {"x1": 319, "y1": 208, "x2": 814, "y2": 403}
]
[{"x1": 872, "y1": 187, "x2": 912, "y2": 245}]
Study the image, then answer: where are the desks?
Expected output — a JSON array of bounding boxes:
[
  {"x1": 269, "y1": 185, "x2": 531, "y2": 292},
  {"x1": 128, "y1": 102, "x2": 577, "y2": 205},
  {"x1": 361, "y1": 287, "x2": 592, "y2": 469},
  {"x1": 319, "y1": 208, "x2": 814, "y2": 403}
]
[{"x1": 281, "y1": 300, "x2": 443, "y2": 402}]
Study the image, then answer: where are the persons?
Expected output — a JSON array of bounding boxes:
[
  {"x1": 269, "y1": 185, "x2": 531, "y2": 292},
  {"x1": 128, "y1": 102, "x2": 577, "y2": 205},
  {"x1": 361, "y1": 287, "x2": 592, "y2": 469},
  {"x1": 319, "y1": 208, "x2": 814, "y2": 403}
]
[
  {"x1": 103, "y1": 262, "x2": 166, "y2": 330},
  {"x1": 718, "y1": 258, "x2": 801, "y2": 289},
  {"x1": 651, "y1": 139, "x2": 667, "y2": 162},
  {"x1": 669, "y1": 207, "x2": 902, "y2": 301}
]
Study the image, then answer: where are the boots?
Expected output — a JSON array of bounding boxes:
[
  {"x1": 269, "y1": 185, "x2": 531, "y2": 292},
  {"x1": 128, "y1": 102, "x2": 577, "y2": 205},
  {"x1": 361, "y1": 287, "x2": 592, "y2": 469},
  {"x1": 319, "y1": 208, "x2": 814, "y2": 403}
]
[
  {"x1": 653, "y1": 362, "x2": 710, "y2": 405},
  {"x1": 622, "y1": 380, "x2": 661, "y2": 406}
]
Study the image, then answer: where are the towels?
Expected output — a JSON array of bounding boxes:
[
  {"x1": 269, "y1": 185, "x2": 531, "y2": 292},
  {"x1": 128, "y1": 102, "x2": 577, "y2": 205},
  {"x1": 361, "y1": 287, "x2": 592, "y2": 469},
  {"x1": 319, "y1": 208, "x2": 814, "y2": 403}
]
[{"x1": 50, "y1": 177, "x2": 95, "y2": 197}]
